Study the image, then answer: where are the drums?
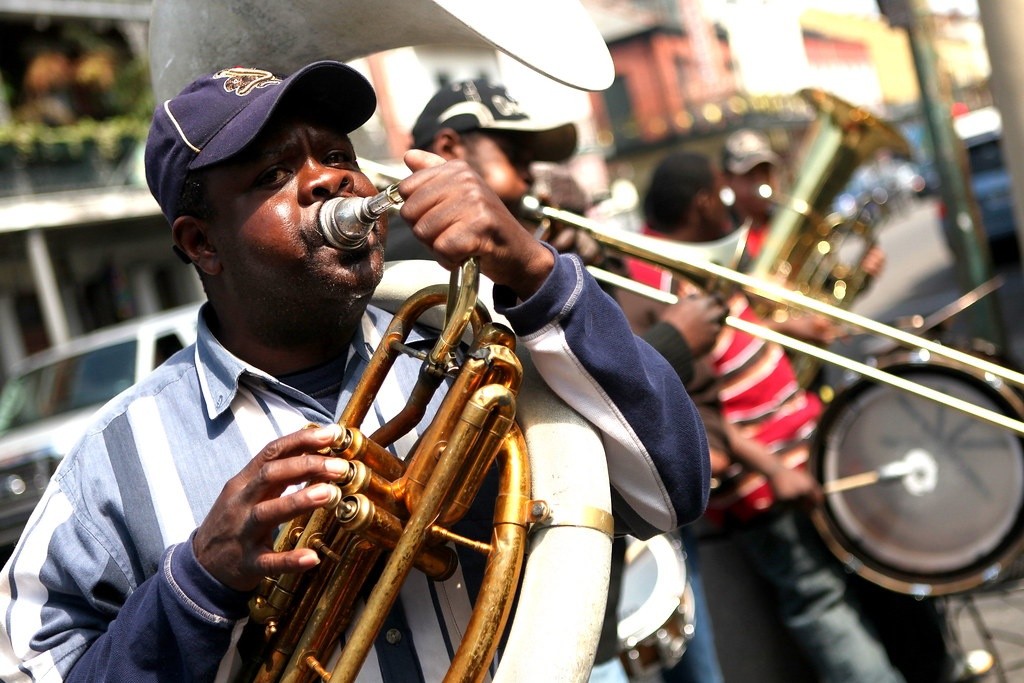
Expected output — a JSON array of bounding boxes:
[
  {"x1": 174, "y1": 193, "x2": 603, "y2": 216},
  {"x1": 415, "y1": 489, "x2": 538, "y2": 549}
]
[
  {"x1": 805, "y1": 345, "x2": 1021, "y2": 602},
  {"x1": 589, "y1": 527, "x2": 700, "y2": 683}
]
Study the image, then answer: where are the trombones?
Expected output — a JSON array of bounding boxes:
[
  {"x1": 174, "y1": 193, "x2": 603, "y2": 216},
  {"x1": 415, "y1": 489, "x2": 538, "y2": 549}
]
[{"x1": 512, "y1": 191, "x2": 1024, "y2": 433}]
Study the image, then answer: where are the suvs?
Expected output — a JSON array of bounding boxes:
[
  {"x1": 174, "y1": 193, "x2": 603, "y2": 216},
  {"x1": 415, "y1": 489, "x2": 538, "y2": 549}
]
[{"x1": 942, "y1": 104, "x2": 1020, "y2": 268}]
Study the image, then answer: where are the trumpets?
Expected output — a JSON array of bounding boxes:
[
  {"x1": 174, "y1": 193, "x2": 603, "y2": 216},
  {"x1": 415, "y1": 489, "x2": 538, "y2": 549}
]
[{"x1": 548, "y1": 210, "x2": 755, "y2": 269}]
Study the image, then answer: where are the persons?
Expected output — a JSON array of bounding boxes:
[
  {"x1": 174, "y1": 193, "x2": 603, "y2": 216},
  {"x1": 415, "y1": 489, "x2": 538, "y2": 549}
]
[
  {"x1": 0, "y1": 60, "x2": 712, "y2": 683},
  {"x1": 618, "y1": 130, "x2": 913, "y2": 680},
  {"x1": 381, "y1": 76, "x2": 733, "y2": 683}
]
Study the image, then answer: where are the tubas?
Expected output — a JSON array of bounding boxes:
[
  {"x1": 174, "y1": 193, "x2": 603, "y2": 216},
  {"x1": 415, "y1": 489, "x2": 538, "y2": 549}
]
[{"x1": 738, "y1": 83, "x2": 916, "y2": 332}]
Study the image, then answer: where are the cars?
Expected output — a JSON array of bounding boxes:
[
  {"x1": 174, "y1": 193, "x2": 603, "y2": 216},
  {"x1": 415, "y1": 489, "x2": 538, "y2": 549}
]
[{"x1": 0, "y1": 302, "x2": 207, "y2": 565}]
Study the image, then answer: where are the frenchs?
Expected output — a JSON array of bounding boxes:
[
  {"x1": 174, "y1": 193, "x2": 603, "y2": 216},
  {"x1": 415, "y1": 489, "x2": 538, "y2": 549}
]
[{"x1": 149, "y1": 0, "x2": 612, "y2": 683}]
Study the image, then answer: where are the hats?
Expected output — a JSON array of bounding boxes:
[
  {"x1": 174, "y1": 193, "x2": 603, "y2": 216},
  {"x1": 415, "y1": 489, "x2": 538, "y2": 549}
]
[
  {"x1": 144, "y1": 60, "x2": 377, "y2": 264},
  {"x1": 412, "y1": 80, "x2": 577, "y2": 164},
  {"x1": 720, "y1": 128, "x2": 782, "y2": 175}
]
